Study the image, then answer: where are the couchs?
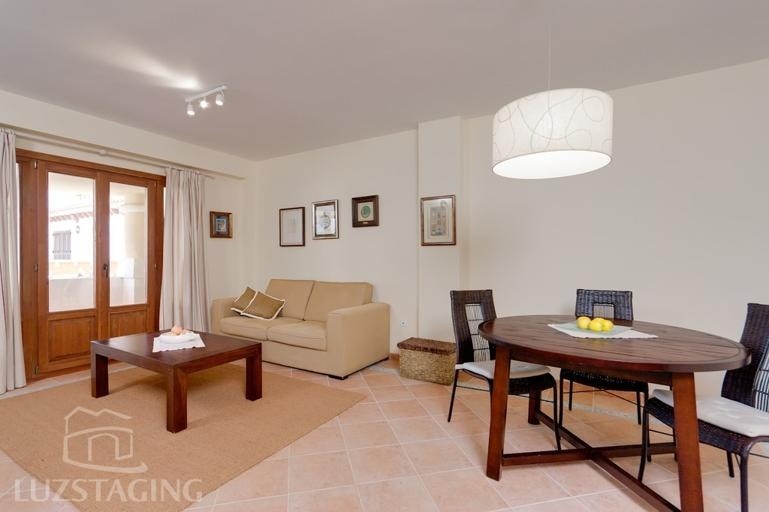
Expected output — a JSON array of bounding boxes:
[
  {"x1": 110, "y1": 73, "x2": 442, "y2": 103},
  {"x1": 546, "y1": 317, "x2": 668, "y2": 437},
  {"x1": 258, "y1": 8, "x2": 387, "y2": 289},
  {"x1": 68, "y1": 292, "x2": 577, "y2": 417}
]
[{"x1": 212, "y1": 279, "x2": 390, "y2": 381}]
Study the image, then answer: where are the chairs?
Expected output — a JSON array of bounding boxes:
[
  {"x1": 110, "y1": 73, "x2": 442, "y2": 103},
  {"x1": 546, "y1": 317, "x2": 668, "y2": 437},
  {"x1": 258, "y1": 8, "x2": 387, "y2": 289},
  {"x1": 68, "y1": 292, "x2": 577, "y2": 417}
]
[
  {"x1": 636, "y1": 302, "x2": 769, "y2": 512},
  {"x1": 448, "y1": 289, "x2": 562, "y2": 453},
  {"x1": 557, "y1": 288, "x2": 653, "y2": 464}
]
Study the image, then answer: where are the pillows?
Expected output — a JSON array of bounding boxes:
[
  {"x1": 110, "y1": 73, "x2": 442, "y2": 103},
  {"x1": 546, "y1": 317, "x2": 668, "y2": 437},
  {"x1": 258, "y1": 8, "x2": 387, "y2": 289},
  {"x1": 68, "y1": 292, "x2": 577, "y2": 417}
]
[
  {"x1": 240, "y1": 290, "x2": 286, "y2": 320},
  {"x1": 230, "y1": 286, "x2": 257, "y2": 314}
]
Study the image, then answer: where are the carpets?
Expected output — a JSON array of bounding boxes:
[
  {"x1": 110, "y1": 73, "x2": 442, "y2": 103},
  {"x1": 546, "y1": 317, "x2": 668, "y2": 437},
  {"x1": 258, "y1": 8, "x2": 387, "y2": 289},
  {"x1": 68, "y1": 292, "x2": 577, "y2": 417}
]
[{"x1": 0, "y1": 361, "x2": 365, "y2": 512}]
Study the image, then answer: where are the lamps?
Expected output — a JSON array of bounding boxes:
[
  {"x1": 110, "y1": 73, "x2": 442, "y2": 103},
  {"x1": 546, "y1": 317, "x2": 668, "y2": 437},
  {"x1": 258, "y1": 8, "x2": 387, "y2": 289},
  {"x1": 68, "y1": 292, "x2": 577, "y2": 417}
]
[
  {"x1": 184, "y1": 85, "x2": 227, "y2": 115},
  {"x1": 490, "y1": 0, "x2": 615, "y2": 180}
]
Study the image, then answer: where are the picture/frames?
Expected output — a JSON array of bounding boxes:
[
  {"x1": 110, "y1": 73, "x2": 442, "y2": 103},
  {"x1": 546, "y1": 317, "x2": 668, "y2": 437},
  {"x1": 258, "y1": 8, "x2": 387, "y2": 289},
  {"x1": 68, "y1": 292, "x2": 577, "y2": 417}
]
[
  {"x1": 209, "y1": 211, "x2": 232, "y2": 238},
  {"x1": 352, "y1": 195, "x2": 379, "y2": 228},
  {"x1": 279, "y1": 206, "x2": 305, "y2": 247},
  {"x1": 420, "y1": 194, "x2": 456, "y2": 246},
  {"x1": 312, "y1": 199, "x2": 339, "y2": 240}
]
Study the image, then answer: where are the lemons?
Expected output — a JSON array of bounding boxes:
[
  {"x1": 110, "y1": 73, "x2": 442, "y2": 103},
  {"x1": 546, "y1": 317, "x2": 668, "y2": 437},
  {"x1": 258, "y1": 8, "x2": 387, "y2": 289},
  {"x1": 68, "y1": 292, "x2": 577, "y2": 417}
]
[
  {"x1": 593, "y1": 318, "x2": 603, "y2": 323},
  {"x1": 600, "y1": 320, "x2": 613, "y2": 331},
  {"x1": 588, "y1": 320, "x2": 601, "y2": 332},
  {"x1": 577, "y1": 316, "x2": 592, "y2": 329}
]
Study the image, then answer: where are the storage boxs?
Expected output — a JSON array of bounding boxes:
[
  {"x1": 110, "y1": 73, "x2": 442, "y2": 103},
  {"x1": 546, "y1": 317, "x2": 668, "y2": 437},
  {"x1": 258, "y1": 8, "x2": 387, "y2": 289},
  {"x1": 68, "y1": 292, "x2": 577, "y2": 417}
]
[{"x1": 397, "y1": 337, "x2": 457, "y2": 385}]
